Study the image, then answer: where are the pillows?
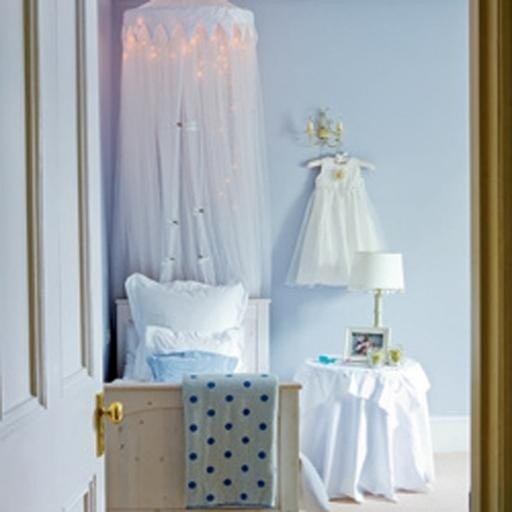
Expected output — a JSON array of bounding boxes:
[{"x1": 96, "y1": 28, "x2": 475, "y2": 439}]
[{"x1": 123, "y1": 272, "x2": 252, "y2": 378}]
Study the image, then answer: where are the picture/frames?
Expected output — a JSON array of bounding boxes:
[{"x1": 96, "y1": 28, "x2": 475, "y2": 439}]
[{"x1": 345, "y1": 328, "x2": 390, "y2": 367}]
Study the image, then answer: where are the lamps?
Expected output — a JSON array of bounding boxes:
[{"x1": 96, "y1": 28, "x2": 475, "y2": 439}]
[{"x1": 349, "y1": 251, "x2": 403, "y2": 328}]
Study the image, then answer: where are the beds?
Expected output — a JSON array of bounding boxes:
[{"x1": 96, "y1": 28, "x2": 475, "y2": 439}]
[{"x1": 104, "y1": 299, "x2": 302, "y2": 512}]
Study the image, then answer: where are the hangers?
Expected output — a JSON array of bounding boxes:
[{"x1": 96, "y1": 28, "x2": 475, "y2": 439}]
[{"x1": 307, "y1": 143, "x2": 375, "y2": 172}]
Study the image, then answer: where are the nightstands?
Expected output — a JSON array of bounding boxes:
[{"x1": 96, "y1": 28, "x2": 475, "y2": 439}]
[{"x1": 301, "y1": 356, "x2": 420, "y2": 499}]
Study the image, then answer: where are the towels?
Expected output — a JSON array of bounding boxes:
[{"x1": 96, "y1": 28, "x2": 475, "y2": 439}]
[{"x1": 182, "y1": 373, "x2": 279, "y2": 510}]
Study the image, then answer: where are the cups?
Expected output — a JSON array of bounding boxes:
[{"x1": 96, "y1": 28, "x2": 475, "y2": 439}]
[
  {"x1": 367, "y1": 346, "x2": 385, "y2": 369},
  {"x1": 387, "y1": 344, "x2": 404, "y2": 366}
]
